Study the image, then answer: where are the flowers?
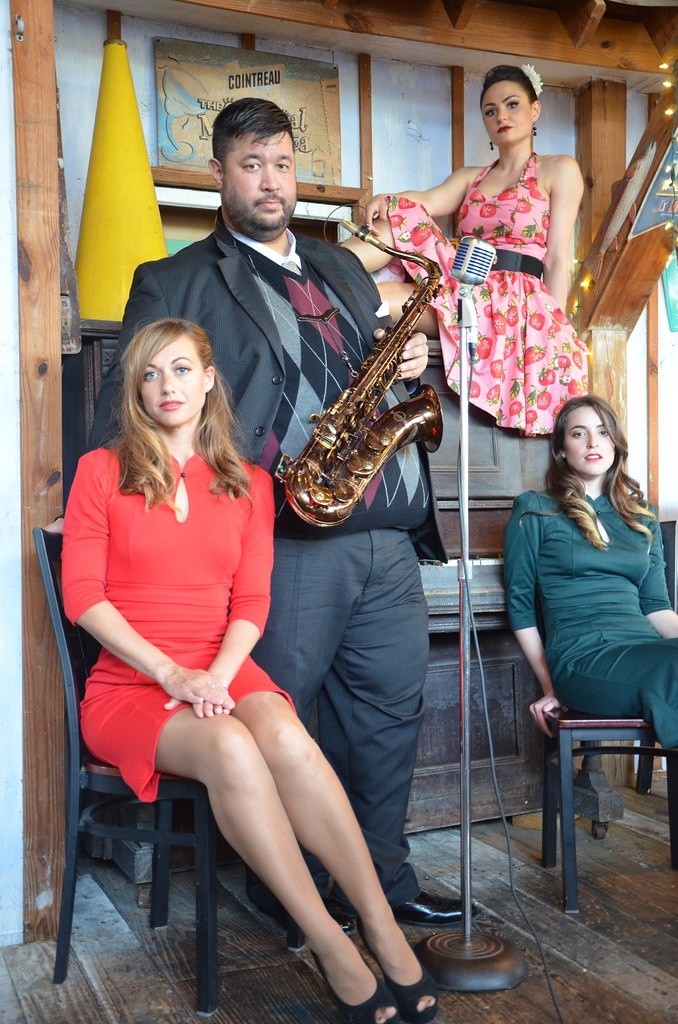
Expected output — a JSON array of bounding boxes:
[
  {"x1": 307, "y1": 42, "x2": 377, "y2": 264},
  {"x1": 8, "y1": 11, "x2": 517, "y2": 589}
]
[{"x1": 521, "y1": 64, "x2": 544, "y2": 97}]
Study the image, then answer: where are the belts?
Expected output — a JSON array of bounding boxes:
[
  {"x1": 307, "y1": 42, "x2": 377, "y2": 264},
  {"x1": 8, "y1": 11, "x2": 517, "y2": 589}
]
[{"x1": 490, "y1": 248, "x2": 543, "y2": 280}]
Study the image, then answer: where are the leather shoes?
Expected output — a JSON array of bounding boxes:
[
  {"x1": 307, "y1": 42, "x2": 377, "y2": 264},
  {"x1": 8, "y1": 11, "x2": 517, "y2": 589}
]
[
  {"x1": 332, "y1": 883, "x2": 479, "y2": 926},
  {"x1": 324, "y1": 908, "x2": 355, "y2": 936}
]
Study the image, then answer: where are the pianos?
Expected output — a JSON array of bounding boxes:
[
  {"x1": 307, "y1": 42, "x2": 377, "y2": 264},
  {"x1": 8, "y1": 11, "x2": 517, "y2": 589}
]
[{"x1": 60, "y1": 317, "x2": 626, "y2": 907}]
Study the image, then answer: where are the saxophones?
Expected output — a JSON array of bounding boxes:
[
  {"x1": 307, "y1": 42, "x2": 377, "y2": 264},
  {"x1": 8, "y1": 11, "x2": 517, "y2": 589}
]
[{"x1": 276, "y1": 219, "x2": 447, "y2": 527}]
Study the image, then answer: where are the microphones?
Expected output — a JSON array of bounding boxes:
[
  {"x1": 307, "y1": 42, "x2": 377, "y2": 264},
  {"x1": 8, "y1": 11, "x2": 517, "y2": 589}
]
[{"x1": 451, "y1": 235, "x2": 497, "y2": 361}]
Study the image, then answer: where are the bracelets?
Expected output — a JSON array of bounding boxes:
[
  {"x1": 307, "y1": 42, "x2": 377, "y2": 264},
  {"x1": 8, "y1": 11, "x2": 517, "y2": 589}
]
[{"x1": 222, "y1": 684, "x2": 228, "y2": 688}]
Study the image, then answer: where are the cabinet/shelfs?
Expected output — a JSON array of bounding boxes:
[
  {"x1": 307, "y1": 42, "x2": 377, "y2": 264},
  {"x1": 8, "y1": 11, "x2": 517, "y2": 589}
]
[{"x1": 75, "y1": 320, "x2": 626, "y2": 909}]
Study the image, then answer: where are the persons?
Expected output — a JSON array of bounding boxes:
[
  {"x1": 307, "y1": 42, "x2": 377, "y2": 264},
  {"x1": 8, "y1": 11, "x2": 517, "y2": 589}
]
[
  {"x1": 63, "y1": 316, "x2": 438, "y2": 1023},
  {"x1": 501, "y1": 395, "x2": 678, "y2": 773},
  {"x1": 89, "y1": 96, "x2": 482, "y2": 946},
  {"x1": 341, "y1": 60, "x2": 589, "y2": 435}
]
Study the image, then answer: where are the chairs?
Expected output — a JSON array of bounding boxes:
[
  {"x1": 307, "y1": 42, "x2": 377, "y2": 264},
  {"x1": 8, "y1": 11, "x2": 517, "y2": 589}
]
[
  {"x1": 33, "y1": 527, "x2": 304, "y2": 1017},
  {"x1": 542, "y1": 521, "x2": 678, "y2": 914}
]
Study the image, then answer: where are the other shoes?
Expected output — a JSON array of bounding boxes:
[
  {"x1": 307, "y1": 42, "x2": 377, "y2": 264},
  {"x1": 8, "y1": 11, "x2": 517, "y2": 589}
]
[
  {"x1": 309, "y1": 940, "x2": 400, "y2": 1024},
  {"x1": 355, "y1": 916, "x2": 439, "y2": 1024}
]
